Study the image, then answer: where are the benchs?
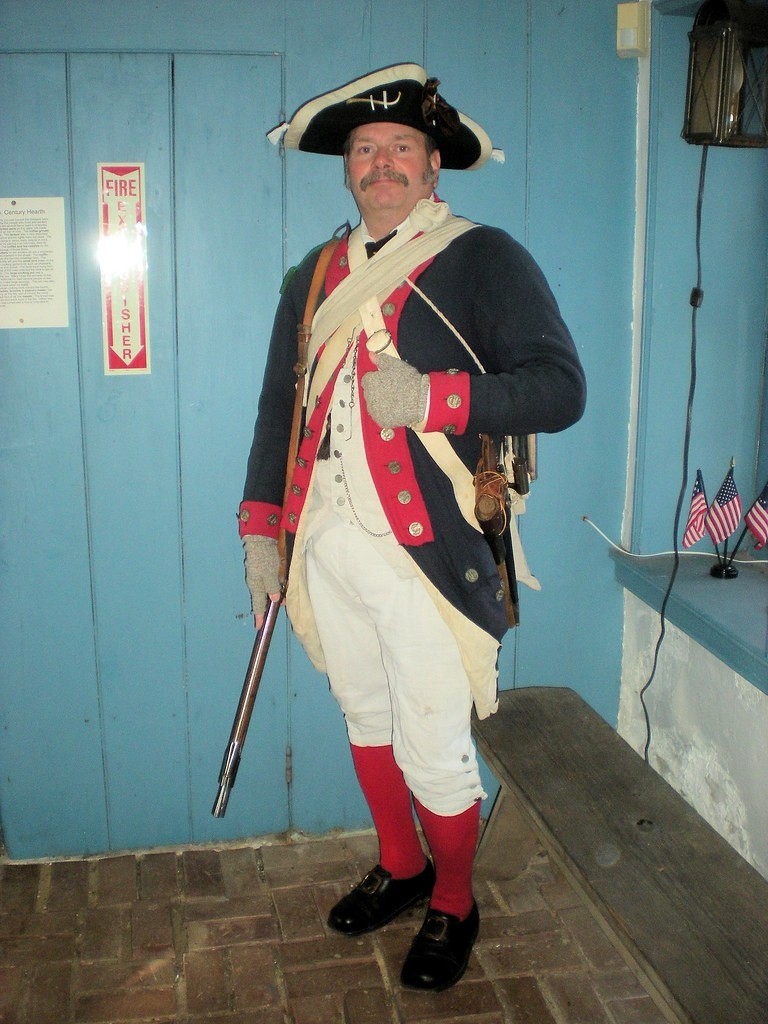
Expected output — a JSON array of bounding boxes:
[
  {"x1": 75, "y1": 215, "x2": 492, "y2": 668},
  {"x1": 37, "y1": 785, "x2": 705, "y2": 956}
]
[{"x1": 470, "y1": 686, "x2": 768, "y2": 1024}]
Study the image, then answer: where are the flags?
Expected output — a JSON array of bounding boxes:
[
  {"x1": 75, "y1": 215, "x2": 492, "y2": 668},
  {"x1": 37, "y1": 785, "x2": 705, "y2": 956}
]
[{"x1": 681, "y1": 471, "x2": 768, "y2": 551}]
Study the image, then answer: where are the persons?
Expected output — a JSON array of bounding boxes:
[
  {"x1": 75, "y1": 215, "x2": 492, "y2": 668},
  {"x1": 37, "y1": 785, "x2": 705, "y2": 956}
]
[{"x1": 236, "y1": 64, "x2": 588, "y2": 990}]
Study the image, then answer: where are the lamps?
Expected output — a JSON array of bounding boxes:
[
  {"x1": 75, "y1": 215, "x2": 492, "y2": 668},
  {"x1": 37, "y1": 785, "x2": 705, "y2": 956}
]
[{"x1": 680, "y1": 0, "x2": 768, "y2": 148}]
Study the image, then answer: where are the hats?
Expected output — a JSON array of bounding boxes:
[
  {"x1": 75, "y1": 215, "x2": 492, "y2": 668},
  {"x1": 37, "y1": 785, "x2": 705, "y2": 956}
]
[{"x1": 268, "y1": 63, "x2": 504, "y2": 170}]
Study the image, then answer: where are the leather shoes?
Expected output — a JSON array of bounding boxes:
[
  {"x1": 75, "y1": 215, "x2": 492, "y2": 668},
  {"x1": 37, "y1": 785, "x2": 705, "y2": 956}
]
[
  {"x1": 401, "y1": 897, "x2": 479, "y2": 990},
  {"x1": 327, "y1": 856, "x2": 433, "y2": 934}
]
